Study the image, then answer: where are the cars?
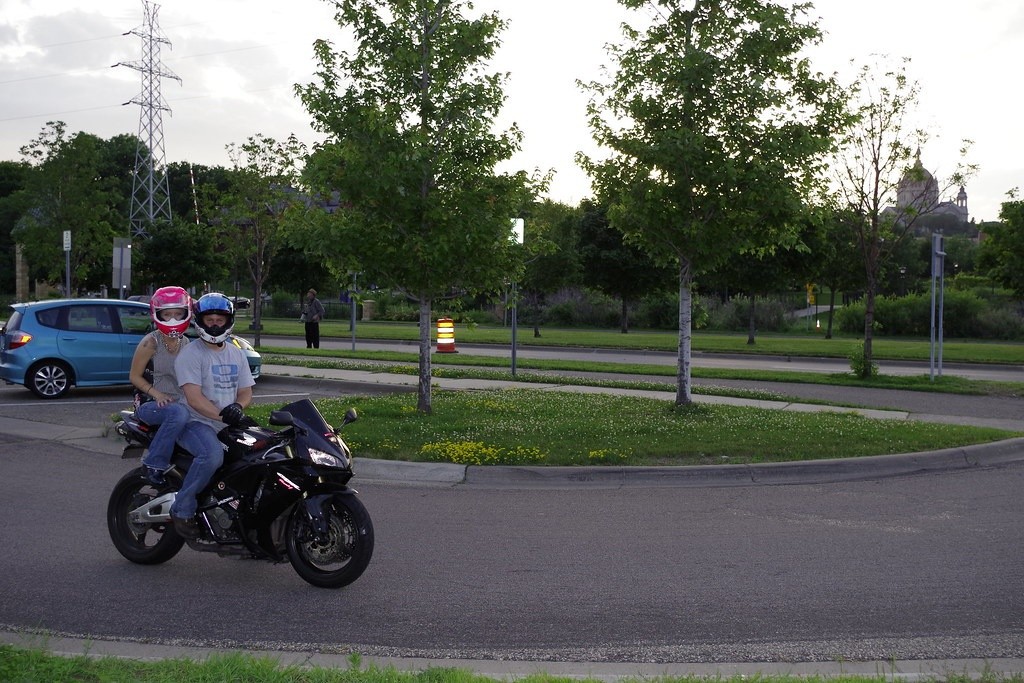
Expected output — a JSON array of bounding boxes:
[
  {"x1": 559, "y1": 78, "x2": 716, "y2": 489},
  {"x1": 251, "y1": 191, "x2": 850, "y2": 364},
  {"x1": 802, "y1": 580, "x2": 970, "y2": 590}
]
[{"x1": 0, "y1": 297, "x2": 263, "y2": 399}]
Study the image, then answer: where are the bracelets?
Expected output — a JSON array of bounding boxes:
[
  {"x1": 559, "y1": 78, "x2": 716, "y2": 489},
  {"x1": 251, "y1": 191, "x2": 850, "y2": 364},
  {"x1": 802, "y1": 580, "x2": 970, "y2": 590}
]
[{"x1": 147, "y1": 386, "x2": 152, "y2": 395}]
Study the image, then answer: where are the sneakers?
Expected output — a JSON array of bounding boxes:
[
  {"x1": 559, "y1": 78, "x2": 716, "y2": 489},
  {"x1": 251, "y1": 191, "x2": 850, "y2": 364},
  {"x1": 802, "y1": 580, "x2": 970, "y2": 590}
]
[
  {"x1": 170, "y1": 509, "x2": 200, "y2": 538},
  {"x1": 145, "y1": 462, "x2": 166, "y2": 484}
]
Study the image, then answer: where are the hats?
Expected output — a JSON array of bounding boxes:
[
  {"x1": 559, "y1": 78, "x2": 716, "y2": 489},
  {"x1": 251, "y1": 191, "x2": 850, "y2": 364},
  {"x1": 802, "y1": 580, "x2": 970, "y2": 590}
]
[{"x1": 307, "y1": 288, "x2": 317, "y2": 296}]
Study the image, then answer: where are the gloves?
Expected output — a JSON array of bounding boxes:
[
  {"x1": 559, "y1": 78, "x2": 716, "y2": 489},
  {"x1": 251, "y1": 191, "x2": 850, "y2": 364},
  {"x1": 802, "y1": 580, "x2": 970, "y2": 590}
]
[
  {"x1": 218, "y1": 402, "x2": 243, "y2": 425},
  {"x1": 239, "y1": 417, "x2": 259, "y2": 429}
]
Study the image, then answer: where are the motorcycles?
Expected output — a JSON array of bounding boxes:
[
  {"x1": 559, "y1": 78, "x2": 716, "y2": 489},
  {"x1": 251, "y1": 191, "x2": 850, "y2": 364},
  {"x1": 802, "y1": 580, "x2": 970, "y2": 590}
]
[{"x1": 103, "y1": 398, "x2": 375, "y2": 590}]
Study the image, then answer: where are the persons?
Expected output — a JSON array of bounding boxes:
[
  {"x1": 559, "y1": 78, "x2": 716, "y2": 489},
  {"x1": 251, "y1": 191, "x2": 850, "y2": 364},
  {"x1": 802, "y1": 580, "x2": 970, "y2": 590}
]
[
  {"x1": 169, "y1": 293, "x2": 256, "y2": 539},
  {"x1": 129, "y1": 286, "x2": 193, "y2": 484},
  {"x1": 305, "y1": 288, "x2": 325, "y2": 349}
]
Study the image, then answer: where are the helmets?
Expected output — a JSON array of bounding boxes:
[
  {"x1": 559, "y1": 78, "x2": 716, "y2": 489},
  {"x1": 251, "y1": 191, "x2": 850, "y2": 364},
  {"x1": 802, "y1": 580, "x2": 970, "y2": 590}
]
[
  {"x1": 150, "y1": 286, "x2": 193, "y2": 338},
  {"x1": 193, "y1": 292, "x2": 235, "y2": 343}
]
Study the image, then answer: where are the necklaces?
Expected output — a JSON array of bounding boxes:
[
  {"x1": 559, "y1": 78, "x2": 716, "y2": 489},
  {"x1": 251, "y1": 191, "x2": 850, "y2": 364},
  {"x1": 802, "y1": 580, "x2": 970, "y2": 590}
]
[{"x1": 159, "y1": 333, "x2": 180, "y2": 353}]
